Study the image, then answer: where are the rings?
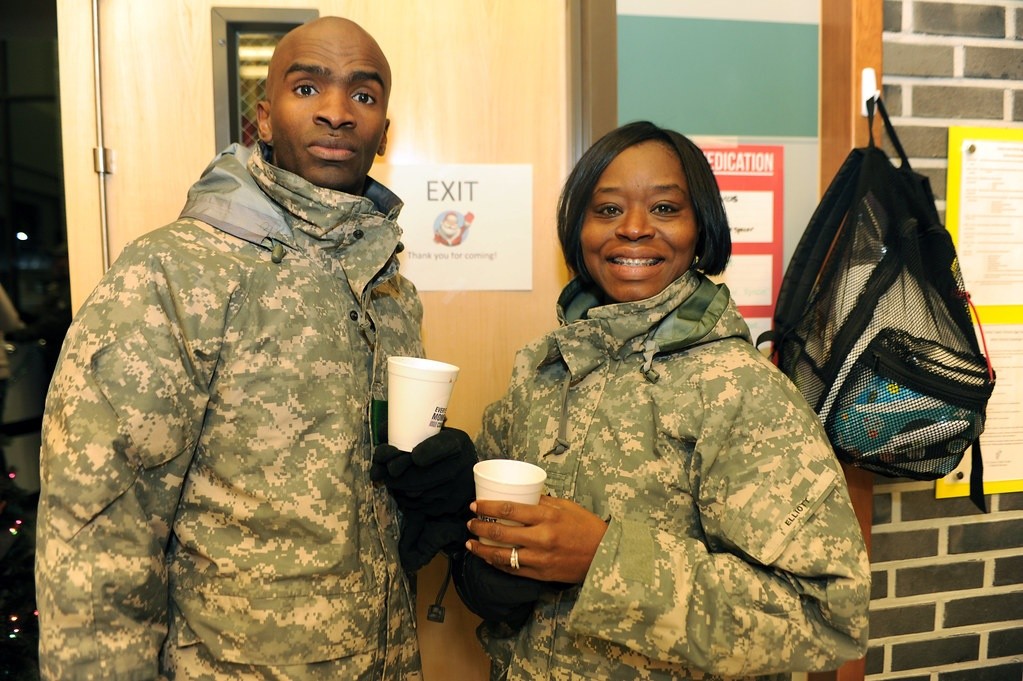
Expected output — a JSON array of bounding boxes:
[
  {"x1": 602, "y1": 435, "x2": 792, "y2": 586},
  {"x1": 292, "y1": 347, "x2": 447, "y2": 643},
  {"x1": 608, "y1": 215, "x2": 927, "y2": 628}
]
[{"x1": 510, "y1": 547, "x2": 520, "y2": 569}]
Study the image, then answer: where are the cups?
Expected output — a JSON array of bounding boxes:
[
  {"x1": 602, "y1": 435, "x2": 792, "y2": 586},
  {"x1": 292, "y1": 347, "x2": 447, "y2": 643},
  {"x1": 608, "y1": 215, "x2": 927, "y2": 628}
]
[
  {"x1": 473, "y1": 459, "x2": 547, "y2": 548},
  {"x1": 387, "y1": 355, "x2": 460, "y2": 453}
]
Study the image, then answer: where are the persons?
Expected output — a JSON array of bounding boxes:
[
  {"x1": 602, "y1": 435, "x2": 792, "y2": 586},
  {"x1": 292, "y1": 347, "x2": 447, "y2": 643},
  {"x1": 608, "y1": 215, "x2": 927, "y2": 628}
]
[
  {"x1": 451, "y1": 121, "x2": 871, "y2": 680},
  {"x1": 35, "y1": 16, "x2": 479, "y2": 681},
  {"x1": 0, "y1": 281, "x2": 27, "y2": 514}
]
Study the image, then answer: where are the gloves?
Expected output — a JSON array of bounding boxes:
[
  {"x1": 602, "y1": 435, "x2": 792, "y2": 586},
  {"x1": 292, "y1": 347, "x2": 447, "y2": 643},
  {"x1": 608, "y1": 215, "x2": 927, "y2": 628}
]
[
  {"x1": 449, "y1": 553, "x2": 554, "y2": 625},
  {"x1": 369, "y1": 420, "x2": 477, "y2": 534}
]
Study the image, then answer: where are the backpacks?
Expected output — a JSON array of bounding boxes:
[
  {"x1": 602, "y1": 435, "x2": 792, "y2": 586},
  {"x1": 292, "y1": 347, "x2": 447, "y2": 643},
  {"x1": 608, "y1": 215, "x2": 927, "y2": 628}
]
[{"x1": 756, "y1": 96, "x2": 997, "y2": 512}]
